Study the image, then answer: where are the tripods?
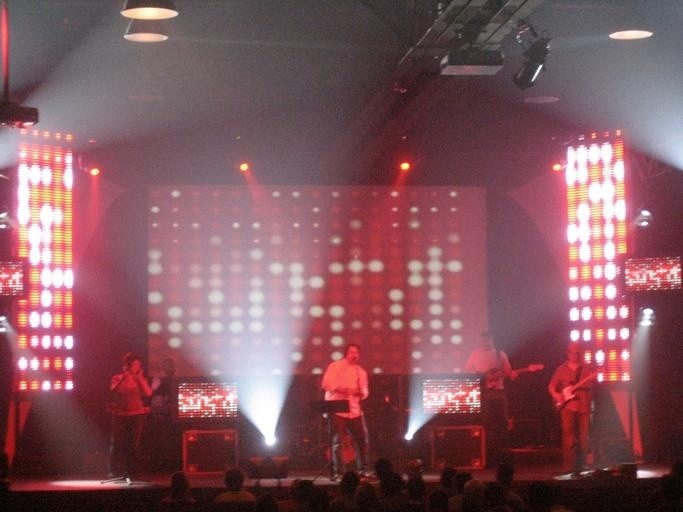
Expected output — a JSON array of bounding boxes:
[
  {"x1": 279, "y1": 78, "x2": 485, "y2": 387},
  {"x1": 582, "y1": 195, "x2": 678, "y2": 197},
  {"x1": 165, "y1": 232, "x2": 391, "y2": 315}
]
[
  {"x1": 310, "y1": 425, "x2": 355, "y2": 480},
  {"x1": 100, "y1": 392, "x2": 151, "y2": 486}
]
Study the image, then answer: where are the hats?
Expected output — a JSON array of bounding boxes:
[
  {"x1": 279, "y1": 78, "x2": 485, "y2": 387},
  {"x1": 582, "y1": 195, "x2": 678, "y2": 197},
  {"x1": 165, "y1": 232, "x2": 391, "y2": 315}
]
[{"x1": 464, "y1": 479, "x2": 484, "y2": 496}]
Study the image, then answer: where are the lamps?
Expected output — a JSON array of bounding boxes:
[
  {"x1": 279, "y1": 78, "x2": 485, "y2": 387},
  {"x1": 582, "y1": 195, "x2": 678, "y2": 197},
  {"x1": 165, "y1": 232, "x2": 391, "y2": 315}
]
[
  {"x1": 119, "y1": 0, "x2": 181, "y2": 45},
  {"x1": 436, "y1": 17, "x2": 554, "y2": 92}
]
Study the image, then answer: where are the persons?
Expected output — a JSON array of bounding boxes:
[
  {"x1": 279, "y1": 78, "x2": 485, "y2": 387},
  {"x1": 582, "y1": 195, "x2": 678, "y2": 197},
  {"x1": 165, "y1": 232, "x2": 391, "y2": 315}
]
[
  {"x1": 546, "y1": 342, "x2": 594, "y2": 478},
  {"x1": 105, "y1": 352, "x2": 185, "y2": 483},
  {"x1": 0, "y1": 451, "x2": 21, "y2": 512},
  {"x1": 462, "y1": 330, "x2": 520, "y2": 474},
  {"x1": 320, "y1": 345, "x2": 370, "y2": 481},
  {"x1": 159, "y1": 459, "x2": 683, "y2": 512}
]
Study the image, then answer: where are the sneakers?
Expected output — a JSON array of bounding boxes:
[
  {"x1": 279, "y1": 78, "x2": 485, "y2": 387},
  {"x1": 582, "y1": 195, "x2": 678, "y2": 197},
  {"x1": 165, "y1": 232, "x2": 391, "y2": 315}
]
[
  {"x1": 331, "y1": 473, "x2": 343, "y2": 482},
  {"x1": 360, "y1": 471, "x2": 378, "y2": 480}
]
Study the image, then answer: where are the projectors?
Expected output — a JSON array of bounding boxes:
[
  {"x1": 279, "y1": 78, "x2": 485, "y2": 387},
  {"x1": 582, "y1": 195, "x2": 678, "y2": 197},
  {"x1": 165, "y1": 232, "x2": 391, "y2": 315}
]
[{"x1": 439, "y1": 50, "x2": 504, "y2": 77}]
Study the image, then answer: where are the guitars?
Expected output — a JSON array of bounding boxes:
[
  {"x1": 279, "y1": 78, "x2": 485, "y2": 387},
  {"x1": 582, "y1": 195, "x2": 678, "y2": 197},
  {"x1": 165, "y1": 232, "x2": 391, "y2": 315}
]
[
  {"x1": 553, "y1": 367, "x2": 599, "y2": 409},
  {"x1": 483, "y1": 363, "x2": 545, "y2": 392}
]
